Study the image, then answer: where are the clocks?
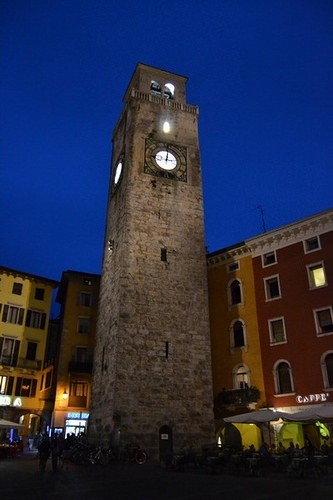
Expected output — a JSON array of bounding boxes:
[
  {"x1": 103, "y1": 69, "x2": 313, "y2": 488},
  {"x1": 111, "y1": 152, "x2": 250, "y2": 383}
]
[{"x1": 143, "y1": 138, "x2": 189, "y2": 183}]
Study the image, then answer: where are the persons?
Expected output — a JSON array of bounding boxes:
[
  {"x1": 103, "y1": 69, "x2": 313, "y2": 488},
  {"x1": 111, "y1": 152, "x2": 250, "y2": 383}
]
[{"x1": 38, "y1": 430, "x2": 333, "y2": 473}]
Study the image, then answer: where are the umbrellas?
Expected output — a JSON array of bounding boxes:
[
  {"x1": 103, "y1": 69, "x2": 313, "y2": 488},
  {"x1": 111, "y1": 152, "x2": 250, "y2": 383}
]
[
  {"x1": 283, "y1": 400, "x2": 333, "y2": 423},
  {"x1": 222, "y1": 409, "x2": 288, "y2": 448},
  {"x1": 0, "y1": 418, "x2": 25, "y2": 430}
]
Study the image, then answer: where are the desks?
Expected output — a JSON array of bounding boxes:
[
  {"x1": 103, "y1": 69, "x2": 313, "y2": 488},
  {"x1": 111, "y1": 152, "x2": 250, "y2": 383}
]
[{"x1": 218, "y1": 448, "x2": 333, "y2": 474}]
